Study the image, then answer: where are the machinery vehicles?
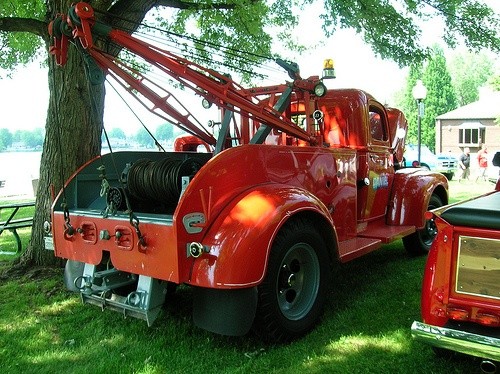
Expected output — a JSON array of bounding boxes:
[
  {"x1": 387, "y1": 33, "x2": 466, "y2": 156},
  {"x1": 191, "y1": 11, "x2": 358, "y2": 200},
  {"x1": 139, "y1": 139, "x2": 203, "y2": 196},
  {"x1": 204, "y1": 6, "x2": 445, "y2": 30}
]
[{"x1": 0, "y1": 0, "x2": 451, "y2": 339}]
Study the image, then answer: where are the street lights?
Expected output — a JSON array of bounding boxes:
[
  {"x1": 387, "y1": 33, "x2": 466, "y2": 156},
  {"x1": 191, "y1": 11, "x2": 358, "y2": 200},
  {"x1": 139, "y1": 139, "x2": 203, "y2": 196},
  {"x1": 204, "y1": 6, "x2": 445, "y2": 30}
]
[{"x1": 411, "y1": 80, "x2": 428, "y2": 167}]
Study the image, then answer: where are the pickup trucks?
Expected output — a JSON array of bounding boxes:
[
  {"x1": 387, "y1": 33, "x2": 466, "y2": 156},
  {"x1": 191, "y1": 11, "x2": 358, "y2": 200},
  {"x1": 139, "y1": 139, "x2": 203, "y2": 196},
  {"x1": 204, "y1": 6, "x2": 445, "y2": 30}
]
[{"x1": 410, "y1": 173, "x2": 499, "y2": 373}]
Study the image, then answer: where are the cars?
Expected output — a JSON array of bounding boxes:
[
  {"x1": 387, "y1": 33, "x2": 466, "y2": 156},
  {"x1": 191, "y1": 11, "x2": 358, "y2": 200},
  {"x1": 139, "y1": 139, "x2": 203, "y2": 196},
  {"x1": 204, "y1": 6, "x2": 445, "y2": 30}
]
[{"x1": 401, "y1": 145, "x2": 456, "y2": 177}]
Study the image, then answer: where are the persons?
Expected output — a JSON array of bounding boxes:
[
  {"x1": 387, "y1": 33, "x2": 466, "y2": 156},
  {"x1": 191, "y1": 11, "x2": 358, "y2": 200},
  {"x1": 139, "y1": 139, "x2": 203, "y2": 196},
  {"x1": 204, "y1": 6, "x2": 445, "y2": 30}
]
[
  {"x1": 459, "y1": 146, "x2": 471, "y2": 183},
  {"x1": 474, "y1": 144, "x2": 488, "y2": 183}
]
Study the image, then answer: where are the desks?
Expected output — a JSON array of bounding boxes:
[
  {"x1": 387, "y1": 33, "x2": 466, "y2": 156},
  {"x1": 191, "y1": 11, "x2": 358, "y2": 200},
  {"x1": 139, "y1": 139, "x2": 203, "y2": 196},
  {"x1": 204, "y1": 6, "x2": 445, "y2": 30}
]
[{"x1": 0, "y1": 202, "x2": 36, "y2": 256}]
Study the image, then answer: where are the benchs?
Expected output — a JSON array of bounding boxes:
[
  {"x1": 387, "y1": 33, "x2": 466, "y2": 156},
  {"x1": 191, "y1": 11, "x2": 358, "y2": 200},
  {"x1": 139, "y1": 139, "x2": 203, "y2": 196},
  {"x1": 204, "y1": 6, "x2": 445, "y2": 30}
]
[{"x1": 0, "y1": 217, "x2": 35, "y2": 230}]
